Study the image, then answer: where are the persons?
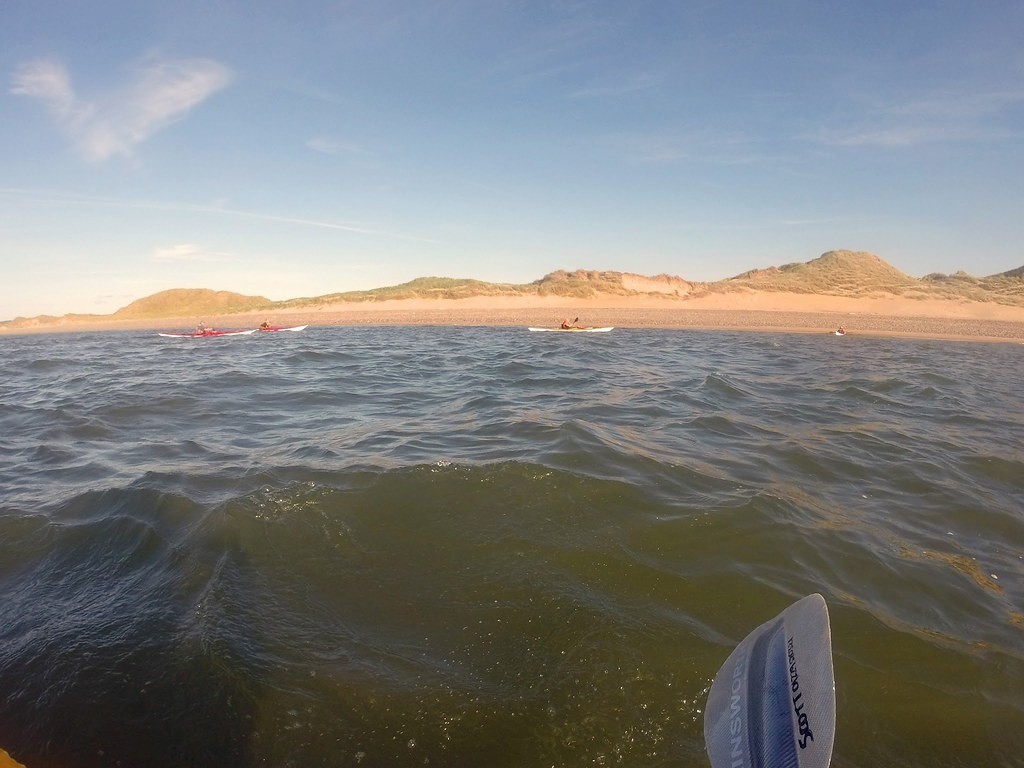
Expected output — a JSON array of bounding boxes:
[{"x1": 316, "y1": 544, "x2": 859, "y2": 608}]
[
  {"x1": 562, "y1": 318, "x2": 571, "y2": 328},
  {"x1": 839, "y1": 326, "x2": 845, "y2": 334},
  {"x1": 196, "y1": 320, "x2": 205, "y2": 334},
  {"x1": 261, "y1": 318, "x2": 271, "y2": 329}
]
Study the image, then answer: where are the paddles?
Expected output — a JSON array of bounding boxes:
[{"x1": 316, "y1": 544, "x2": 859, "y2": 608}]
[
  {"x1": 703, "y1": 592, "x2": 836, "y2": 768},
  {"x1": 568, "y1": 316, "x2": 579, "y2": 327}
]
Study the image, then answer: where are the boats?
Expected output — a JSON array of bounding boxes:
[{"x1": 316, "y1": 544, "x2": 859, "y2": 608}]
[
  {"x1": 830, "y1": 330, "x2": 846, "y2": 337},
  {"x1": 529, "y1": 326, "x2": 614, "y2": 332},
  {"x1": 158, "y1": 324, "x2": 309, "y2": 338}
]
